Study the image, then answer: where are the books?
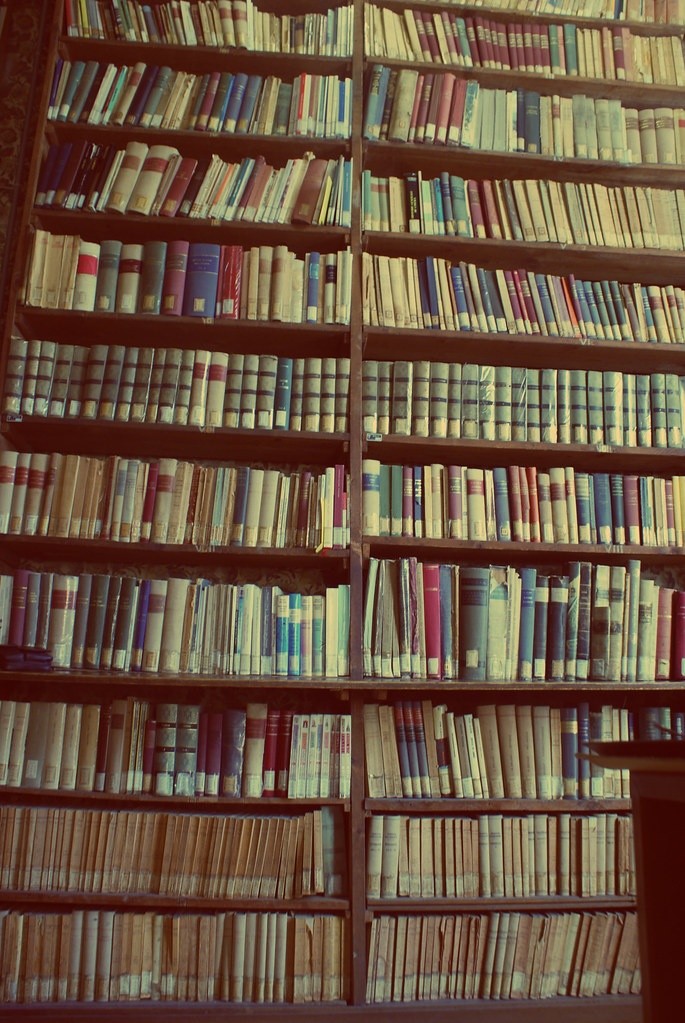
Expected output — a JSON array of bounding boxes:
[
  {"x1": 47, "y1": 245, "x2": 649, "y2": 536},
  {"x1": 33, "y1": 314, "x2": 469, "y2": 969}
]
[{"x1": 0, "y1": 1, "x2": 685, "y2": 1004}]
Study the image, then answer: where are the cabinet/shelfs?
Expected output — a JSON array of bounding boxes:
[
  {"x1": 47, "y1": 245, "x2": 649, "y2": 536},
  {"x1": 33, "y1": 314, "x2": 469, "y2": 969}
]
[{"x1": 0, "y1": 2, "x2": 685, "y2": 1023}]
